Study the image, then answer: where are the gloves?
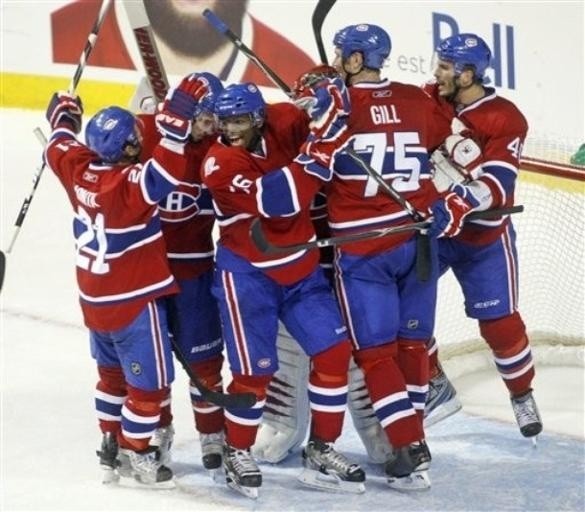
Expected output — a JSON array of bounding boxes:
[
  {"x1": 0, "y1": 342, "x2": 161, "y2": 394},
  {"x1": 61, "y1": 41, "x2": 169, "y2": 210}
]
[
  {"x1": 155, "y1": 76, "x2": 210, "y2": 142},
  {"x1": 426, "y1": 182, "x2": 480, "y2": 239},
  {"x1": 292, "y1": 65, "x2": 355, "y2": 183},
  {"x1": 46, "y1": 91, "x2": 83, "y2": 134}
]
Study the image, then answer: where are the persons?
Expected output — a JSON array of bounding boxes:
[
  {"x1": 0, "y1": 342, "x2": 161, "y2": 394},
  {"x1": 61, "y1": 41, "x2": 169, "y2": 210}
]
[
  {"x1": 50, "y1": 0, "x2": 317, "y2": 89},
  {"x1": 133, "y1": 72, "x2": 225, "y2": 469},
  {"x1": 199, "y1": 73, "x2": 365, "y2": 487},
  {"x1": 424, "y1": 33, "x2": 542, "y2": 438},
  {"x1": 327, "y1": 23, "x2": 484, "y2": 477},
  {"x1": 42, "y1": 72, "x2": 214, "y2": 484},
  {"x1": 250, "y1": 65, "x2": 395, "y2": 465}
]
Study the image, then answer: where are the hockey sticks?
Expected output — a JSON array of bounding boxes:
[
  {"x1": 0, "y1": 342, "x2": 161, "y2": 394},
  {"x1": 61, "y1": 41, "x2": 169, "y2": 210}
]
[
  {"x1": 173, "y1": 343, "x2": 257, "y2": 409},
  {"x1": 249, "y1": 206, "x2": 525, "y2": 256},
  {"x1": 203, "y1": 10, "x2": 430, "y2": 284},
  {"x1": 311, "y1": 2, "x2": 337, "y2": 65}
]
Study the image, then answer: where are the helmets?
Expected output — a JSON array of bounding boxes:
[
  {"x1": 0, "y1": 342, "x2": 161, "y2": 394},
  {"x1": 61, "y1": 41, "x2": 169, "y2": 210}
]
[
  {"x1": 189, "y1": 72, "x2": 266, "y2": 135},
  {"x1": 85, "y1": 105, "x2": 145, "y2": 163},
  {"x1": 436, "y1": 33, "x2": 491, "y2": 80},
  {"x1": 333, "y1": 24, "x2": 391, "y2": 69}
]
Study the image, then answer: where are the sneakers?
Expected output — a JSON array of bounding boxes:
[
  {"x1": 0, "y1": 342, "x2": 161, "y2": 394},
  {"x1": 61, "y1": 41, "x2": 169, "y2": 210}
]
[
  {"x1": 223, "y1": 439, "x2": 262, "y2": 487},
  {"x1": 385, "y1": 438, "x2": 431, "y2": 478},
  {"x1": 97, "y1": 424, "x2": 173, "y2": 484},
  {"x1": 198, "y1": 428, "x2": 224, "y2": 469},
  {"x1": 302, "y1": 436, "x2": 365, "y2": 482},
  {"x1": 423, "y1": 370, "x2": 456, "y2": 421},
  {"x1": 510, "y1": 388, "x2": 543, "y2": 437}
]
